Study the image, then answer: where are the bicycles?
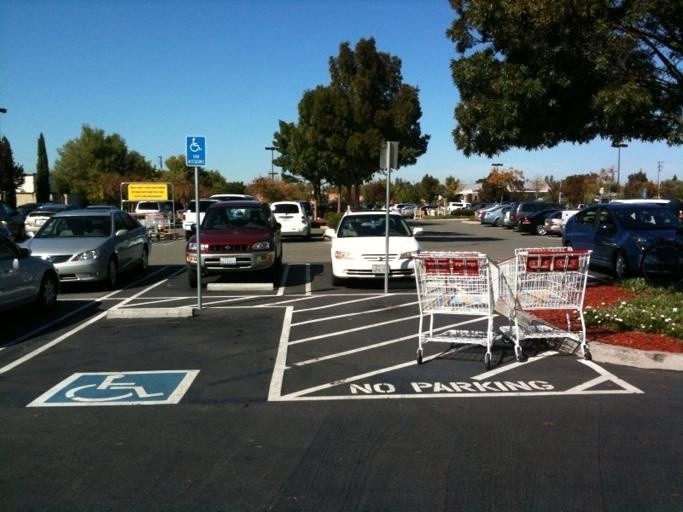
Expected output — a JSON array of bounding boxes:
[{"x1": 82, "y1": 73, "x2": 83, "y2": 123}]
[{"x1": 640, "y1": 230, "x2": 682, "y2": 292}]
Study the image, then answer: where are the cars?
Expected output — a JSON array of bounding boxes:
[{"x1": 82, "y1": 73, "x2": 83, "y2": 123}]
[
  {"x1": 20, "y1": 208, "x2": 151, "y2": 290},
  {"x1": 324, "y1": 205, "x2": 423, "y2": 286},
  {"x1": 0, "y1": 231, "x2": 59, "y2": 317},
  {"x1": 1, "y1": 197, "x2": 179, "y2": 247},
  {"x1": 181, "y1": 193, "x2": 314, "y2": 242},
  {"x1": 559, "y1": 204, "x2": 681, "y2": 282}
]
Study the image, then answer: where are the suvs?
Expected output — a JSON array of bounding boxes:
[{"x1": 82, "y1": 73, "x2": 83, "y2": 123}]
[{"x1": 184, "y1": 200, "x2": 283, "y2": 289}]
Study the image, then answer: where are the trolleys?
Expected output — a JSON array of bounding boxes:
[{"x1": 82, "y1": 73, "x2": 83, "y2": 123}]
[
  {"x1": 496, "y1": 246, "x2": 593, "y2": 364},
  {"x1": 399, "y1": 249, "x2": 499, "y2": 369}
]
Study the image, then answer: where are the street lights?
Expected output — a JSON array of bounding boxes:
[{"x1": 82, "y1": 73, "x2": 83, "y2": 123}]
[
  {"x1": 491, "y1": 163, "x2": 504, "y2": 173},
  {"x1": 265, "y1": 146, "x2": 282, "y2": 183},
  {"x1": 610, "y1": 143, "x2": 628, "y2": 197}
]
